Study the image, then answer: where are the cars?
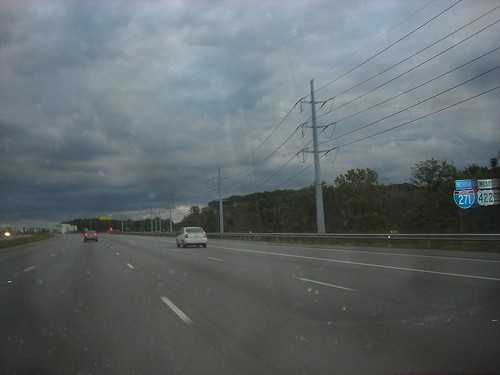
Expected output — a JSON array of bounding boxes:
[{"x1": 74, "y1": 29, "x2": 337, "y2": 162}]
[
  {"x1": 84, "y1": 231, "x2": 98, "y2": 242},
  {"x1": 176, "y1": 227, "x2": 207, "y2": 248}
]
